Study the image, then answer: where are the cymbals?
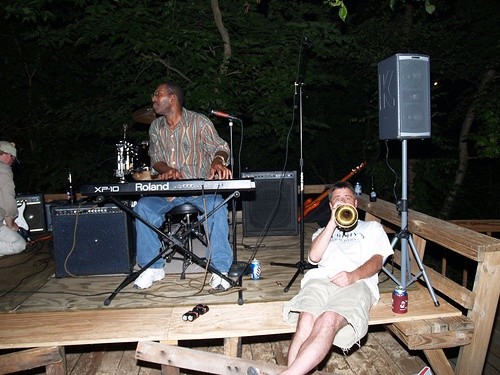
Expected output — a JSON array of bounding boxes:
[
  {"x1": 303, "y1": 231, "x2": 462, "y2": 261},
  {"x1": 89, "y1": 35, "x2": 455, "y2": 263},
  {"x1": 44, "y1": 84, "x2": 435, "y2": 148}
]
[{"x1": 132, "y1": 107, "x2": 157, "y2": 122}]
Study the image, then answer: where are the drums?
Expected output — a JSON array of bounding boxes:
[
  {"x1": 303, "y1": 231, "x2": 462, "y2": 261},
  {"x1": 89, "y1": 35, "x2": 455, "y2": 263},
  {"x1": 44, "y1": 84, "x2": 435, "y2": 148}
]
[{"x1": 131, "y1": 140, "x2": 152, "y2": 181}]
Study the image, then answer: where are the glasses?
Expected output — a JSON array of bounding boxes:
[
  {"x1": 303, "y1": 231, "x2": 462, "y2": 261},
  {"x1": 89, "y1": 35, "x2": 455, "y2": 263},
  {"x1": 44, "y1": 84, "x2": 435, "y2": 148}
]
[{"x1": 150, "y1": 92, "x2": 175, "y2": 98}]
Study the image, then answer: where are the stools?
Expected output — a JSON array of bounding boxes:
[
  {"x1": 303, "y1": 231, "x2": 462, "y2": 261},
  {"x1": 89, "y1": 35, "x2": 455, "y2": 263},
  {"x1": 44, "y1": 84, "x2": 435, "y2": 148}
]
[{"x1": 166, "y1": 203, "x2": 208, "y2": 281}]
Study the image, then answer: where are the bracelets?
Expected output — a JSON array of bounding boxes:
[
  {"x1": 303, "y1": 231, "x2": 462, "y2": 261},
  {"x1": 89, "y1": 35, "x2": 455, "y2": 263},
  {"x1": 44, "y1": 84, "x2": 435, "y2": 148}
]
[{"x1": 215, "y1": 155, "x2": 226, "y2": 166}]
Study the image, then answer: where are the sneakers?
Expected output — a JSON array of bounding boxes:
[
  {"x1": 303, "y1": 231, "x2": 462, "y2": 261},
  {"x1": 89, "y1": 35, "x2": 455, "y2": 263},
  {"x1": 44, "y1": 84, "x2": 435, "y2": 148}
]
[
  {"x1": 212, "y1": 272, "x2": 230, "y2": 291},
  {"x1": 134, "y1": 268, "x2": 166, "y2": 289}
]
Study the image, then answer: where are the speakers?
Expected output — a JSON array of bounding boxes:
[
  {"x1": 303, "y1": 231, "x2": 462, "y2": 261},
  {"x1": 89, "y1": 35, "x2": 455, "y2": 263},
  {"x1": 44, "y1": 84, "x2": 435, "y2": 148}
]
[
  {"x1": 241, "y1": 170, "x2": 300, "y2": 236},
  {"x1": 377, "y1": 53, "x2": 431, "y2": 140},
  {"x1": 14, "y1": 194, "x2": 133, "y2": 278}
]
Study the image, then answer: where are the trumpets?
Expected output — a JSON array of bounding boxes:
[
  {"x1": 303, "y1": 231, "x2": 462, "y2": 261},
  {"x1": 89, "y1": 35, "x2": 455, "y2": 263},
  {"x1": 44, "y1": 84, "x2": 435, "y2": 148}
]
[{"x1": 334, "y1": 204, "x2": 358, "y2": 229}]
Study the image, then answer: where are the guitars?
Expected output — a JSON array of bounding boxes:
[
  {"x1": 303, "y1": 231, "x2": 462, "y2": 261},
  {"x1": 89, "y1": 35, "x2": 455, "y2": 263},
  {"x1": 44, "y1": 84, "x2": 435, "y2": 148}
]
[{"x1": 298, "y1": 161, "x2": 367, "y2": 222}]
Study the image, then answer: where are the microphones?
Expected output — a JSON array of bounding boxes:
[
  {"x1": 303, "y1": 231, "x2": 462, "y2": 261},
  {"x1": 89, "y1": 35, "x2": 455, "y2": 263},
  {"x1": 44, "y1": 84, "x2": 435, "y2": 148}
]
[{"x1": 206, "y1": 106, "x2": 237, "y2": 120}]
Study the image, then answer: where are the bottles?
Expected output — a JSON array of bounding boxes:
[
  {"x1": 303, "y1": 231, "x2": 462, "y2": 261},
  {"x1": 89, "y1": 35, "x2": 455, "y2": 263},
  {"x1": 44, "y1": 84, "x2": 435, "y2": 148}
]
[
  {"x1": 355, "y1": 182, "x2": 362, "y2": 197},
  {"x1": 369, "y1": 175, "x2": 376, "y2": 202},
  {"x1": 65, "y1": 170, "x2": 77, "y2": 206}
]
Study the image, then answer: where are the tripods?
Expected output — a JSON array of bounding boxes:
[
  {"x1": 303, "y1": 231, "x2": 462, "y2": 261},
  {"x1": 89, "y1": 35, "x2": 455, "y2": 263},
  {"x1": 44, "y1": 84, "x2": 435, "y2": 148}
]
[
  {"x1": 374, "y1": 140, "x2": 439, "y2": 308},
  {"x1": 271, "y1": 89, "x2": 318, "y2": 291}
]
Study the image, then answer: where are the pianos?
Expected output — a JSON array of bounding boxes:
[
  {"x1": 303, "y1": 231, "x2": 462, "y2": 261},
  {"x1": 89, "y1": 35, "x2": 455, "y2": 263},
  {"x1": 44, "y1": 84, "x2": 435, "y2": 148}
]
[{"x1": 81, "y1": 176, "x2": 256, "y2": 195}]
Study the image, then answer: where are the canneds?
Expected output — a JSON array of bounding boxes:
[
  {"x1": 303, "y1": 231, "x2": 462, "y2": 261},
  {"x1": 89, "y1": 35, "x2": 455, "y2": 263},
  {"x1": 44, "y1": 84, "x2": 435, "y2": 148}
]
[
  {"x1": 251, "y1": 259, "x2": 261, "y2": 280},
  {"x1": 67, "y1": 167, "x2": 76, "y2": 206},
  {"x1": 391, "y1": 287, "x2": 408, "y2": 313}
]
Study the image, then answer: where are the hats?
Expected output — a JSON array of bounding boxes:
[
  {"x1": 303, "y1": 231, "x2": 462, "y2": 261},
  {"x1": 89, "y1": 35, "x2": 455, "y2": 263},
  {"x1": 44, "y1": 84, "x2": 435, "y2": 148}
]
[{"x1": 0, "y1": 141, "x2": 20, "y2": 165}]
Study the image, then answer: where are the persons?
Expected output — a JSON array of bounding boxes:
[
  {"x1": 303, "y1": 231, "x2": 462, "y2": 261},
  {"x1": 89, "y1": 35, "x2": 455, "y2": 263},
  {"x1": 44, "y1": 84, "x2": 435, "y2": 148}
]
[
  {"x1": 0, "y1": 141, "x2": 27, "y2": 258},
  {"x1": 278, "y1": 181, "x2": 395, "y2": 375},
  {"x1": 132, "y1": 80, "x2": 237, "y2": 295}
]
